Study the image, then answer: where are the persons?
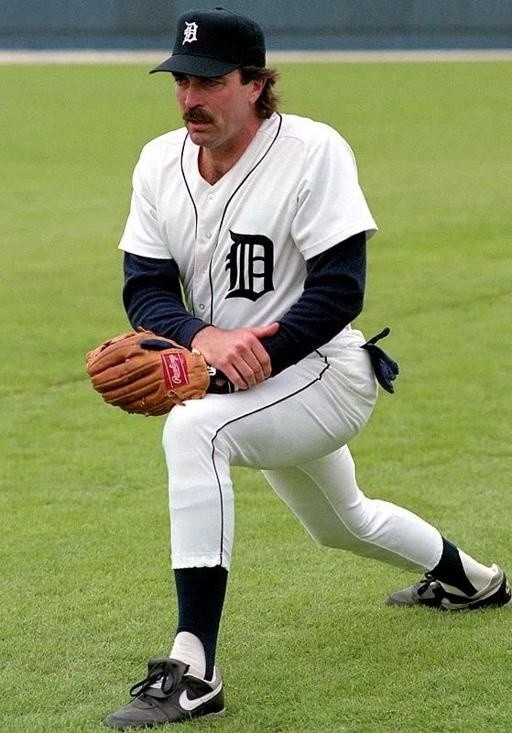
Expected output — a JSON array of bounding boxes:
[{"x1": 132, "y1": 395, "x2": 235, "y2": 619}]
[{"x1": 85, "y1": 7, "x2": 511, "y2": 731}]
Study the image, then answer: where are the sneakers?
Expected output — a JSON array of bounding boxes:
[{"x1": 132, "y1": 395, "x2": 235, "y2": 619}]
[
  {"x1": 384, "y1": 563, "x2": 512, "y2": 612},
  {"x1": 106, "y1": 658, "x2": 226, "y2": 732}
]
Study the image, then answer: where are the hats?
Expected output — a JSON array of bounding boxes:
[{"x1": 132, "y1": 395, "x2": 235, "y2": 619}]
[{"x1": 147, "y1": 6, "x2": 266, "y2": 78}]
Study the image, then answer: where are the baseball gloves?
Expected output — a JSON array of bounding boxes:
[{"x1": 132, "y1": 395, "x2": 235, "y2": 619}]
[{"x1": 85, "y1": 331, "x2": 210, "y2": 415}]
